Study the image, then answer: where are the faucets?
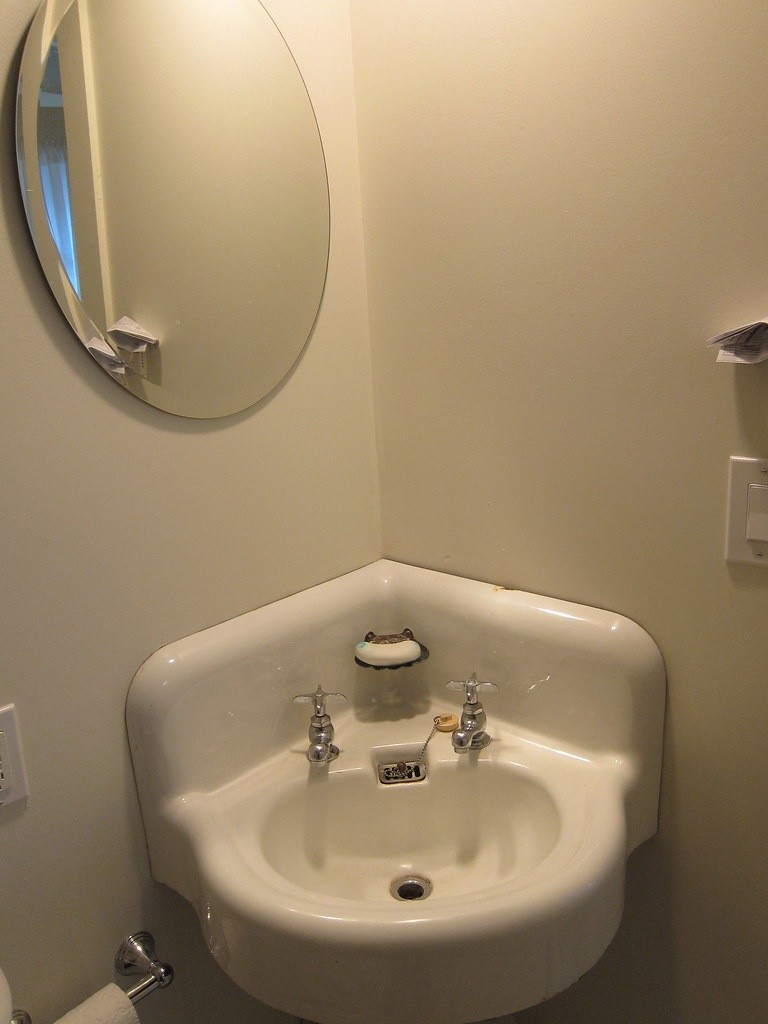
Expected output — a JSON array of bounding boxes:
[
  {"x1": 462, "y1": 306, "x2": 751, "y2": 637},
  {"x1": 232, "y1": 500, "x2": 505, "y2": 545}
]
[
  {"x1": 443, "y1": 670, "x2": 500, "y2": 755},
  {"x1": 292, "y1": 685, "x2": 351, "y2": 765}
]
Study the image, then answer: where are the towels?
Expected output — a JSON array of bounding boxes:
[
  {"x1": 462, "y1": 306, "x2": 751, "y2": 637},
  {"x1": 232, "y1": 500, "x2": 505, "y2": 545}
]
[{"x1": 53, "y1": 981, "x2": 141, "y2": 1024}]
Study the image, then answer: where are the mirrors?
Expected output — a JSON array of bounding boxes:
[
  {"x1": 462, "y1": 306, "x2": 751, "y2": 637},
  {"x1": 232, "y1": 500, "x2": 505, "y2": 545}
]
[{"x1": 14, "y1": 1, "x2": 329, "y2": 420}]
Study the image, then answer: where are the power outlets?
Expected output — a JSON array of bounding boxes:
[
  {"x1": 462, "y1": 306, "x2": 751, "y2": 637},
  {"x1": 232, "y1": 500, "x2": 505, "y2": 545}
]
[{"x1": 0, "y1": 702, "x2": 31, "y2": 810}]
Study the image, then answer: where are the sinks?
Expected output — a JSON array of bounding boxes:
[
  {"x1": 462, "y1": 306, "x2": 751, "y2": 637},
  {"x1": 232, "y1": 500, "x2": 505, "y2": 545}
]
[{"x1": 200, "y1": 735, "x2": 627, "y2": 1024}]
